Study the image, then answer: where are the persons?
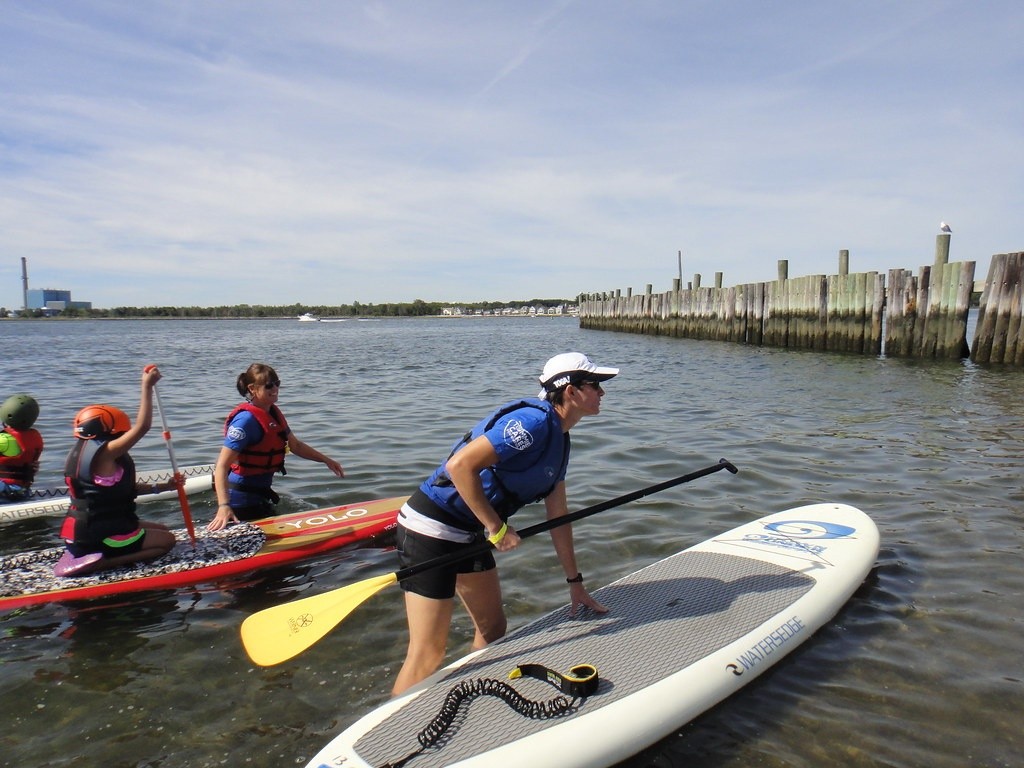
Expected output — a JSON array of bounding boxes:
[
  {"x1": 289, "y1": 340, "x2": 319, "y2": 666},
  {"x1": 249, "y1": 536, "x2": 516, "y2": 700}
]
[
  {"x1": 0, "y1": 395, "x2": 42, "y2": 500},
  {"x1": 388, "y1": 348, "x2": 621, "y2": 702},
  {"x1": 206, "y1": 363, "x2": 347, "y2": 533},
  {"x1": 52, "y1": 364, "x2": 187, "y2": 577}
]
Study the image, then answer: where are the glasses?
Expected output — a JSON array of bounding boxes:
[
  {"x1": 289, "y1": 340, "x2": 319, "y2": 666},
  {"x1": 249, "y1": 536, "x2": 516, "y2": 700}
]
[
  {"x1": 254, "y1": 380, "x2": 281, "y2": 389},
  {"x1": 581, "y1": 381, "x2": 599, "y2": 389}
]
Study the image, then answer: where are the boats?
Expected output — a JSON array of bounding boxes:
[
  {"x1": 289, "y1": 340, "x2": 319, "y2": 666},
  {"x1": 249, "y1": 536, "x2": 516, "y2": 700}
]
[{"x1": 298, "y1": 312, "x2": 320, "y2": 321}]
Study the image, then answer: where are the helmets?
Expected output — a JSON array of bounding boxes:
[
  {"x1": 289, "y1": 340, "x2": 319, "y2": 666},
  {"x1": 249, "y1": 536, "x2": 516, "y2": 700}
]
[
  {"x1": 0, "y1": 395, "x2": 40, "y2": 430},
  {"x1": 72, "y1": 405, "x2": 132, "y2": 440}
]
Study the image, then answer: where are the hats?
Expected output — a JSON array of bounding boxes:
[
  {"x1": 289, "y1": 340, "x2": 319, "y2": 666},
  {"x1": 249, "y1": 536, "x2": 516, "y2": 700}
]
[{"x1": 538, "y1": 352, "x2": 619, "y2": 401}]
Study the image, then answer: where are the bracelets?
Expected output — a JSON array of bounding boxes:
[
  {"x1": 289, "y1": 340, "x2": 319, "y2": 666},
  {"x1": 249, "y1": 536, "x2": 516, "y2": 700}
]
[
  {"x1": 566, "y1": 573, "x2": 584, "y2": 584},
  {"x1": 487, "y1": 522, "x2": 507, "y2": 545},
  {"x1": 217, "y1": 502, "x2": 231, "y2": 508}
]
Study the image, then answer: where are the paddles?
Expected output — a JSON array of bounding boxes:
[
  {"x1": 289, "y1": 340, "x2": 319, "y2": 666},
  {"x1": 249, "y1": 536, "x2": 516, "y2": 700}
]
[
  {"x1": 144, "y1": 364, "x2": 196, "y2": 551},
  {"x1": 240, "y1": 456, "x2": 740, "y2": 668}
]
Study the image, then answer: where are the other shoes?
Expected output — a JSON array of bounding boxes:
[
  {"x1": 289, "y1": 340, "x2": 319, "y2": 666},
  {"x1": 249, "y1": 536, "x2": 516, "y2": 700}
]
[{"x1": 54, "y1": 552, "x2": 105, "y2": 577}]
